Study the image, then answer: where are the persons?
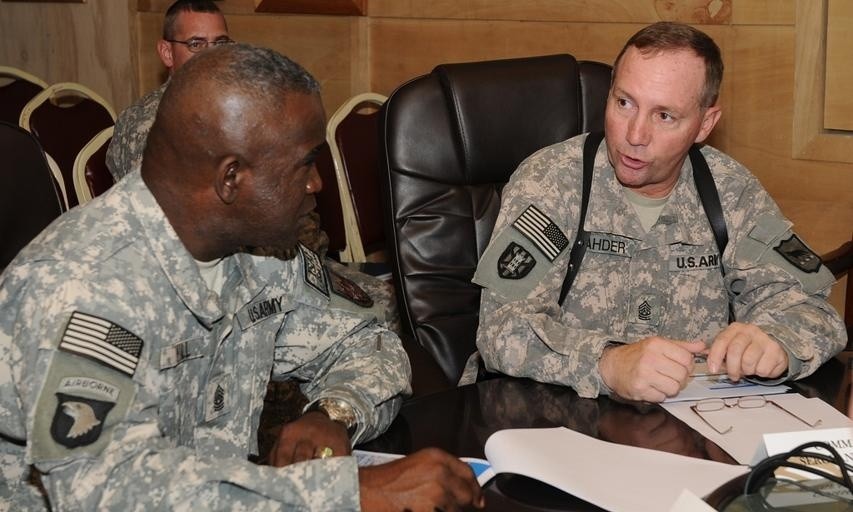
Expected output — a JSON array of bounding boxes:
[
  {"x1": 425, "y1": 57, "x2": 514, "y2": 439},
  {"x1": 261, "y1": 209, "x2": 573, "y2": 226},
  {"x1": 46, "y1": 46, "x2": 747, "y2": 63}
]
[
  {"x1": 105, "y1": 2, "x2": 330, "y2": 259},
  {"x1": 457, "y1": 22, "x2": 849, "y2": 405},
  {"x1": 1, "y1": 41, "x2": 487, "y2": 511}
]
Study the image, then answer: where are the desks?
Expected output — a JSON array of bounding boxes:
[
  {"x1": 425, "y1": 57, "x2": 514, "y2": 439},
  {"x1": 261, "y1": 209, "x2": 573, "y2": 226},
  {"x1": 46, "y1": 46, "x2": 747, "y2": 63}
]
[{"x1": 256, "y1": 353, "x2": 853, "y2": 510}]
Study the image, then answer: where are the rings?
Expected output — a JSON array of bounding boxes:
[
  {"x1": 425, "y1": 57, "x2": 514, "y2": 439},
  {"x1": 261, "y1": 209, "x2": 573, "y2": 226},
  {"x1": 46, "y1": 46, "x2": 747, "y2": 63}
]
[{"x1": 313, "y1": 446, "x2": 334, "y2": 460}]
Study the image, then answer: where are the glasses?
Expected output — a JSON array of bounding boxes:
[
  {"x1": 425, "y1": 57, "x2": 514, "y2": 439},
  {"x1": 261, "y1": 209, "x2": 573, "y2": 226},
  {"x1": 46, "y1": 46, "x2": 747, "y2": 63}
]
[
  {"x1": 690, "y1": 395, "x2": 822, "y2": 435},
  {"x1": 169, "y1": 38, "x2": 236, "y2": 53}
]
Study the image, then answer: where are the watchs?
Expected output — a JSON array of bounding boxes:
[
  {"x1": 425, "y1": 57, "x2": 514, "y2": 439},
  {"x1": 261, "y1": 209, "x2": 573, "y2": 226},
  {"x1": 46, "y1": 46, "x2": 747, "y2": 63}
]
[{"x1": 305, "y1": 397, "x2": 358, "y2": 438}]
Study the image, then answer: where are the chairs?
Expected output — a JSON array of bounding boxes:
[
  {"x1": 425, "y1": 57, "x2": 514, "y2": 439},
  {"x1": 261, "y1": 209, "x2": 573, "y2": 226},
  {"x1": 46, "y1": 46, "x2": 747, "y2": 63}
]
[
  {"x1": 326, "y1": 92, "x2": 389, "y2": 281},
  {"x1": 376, "y1": 54, "x2": 614, "y2": 386},
  {"x1": 0, "y1": 120, "x2": 67, "y2": 277},
  {"x1": 19, "y1": 81, "x2": 117, "y2": 211},
  {"x1": 0, "y1": 66, "x2": 50, "y2": 129},
  {"x1": 73, "y1": 125, "x2": 115, "y2": 208},
  {"x1": 819, "y1": 239, "x2": 852, "y2": 352},
  {"x1": 310, "y1": 129, "x2": 353, "y2": 265}
]
